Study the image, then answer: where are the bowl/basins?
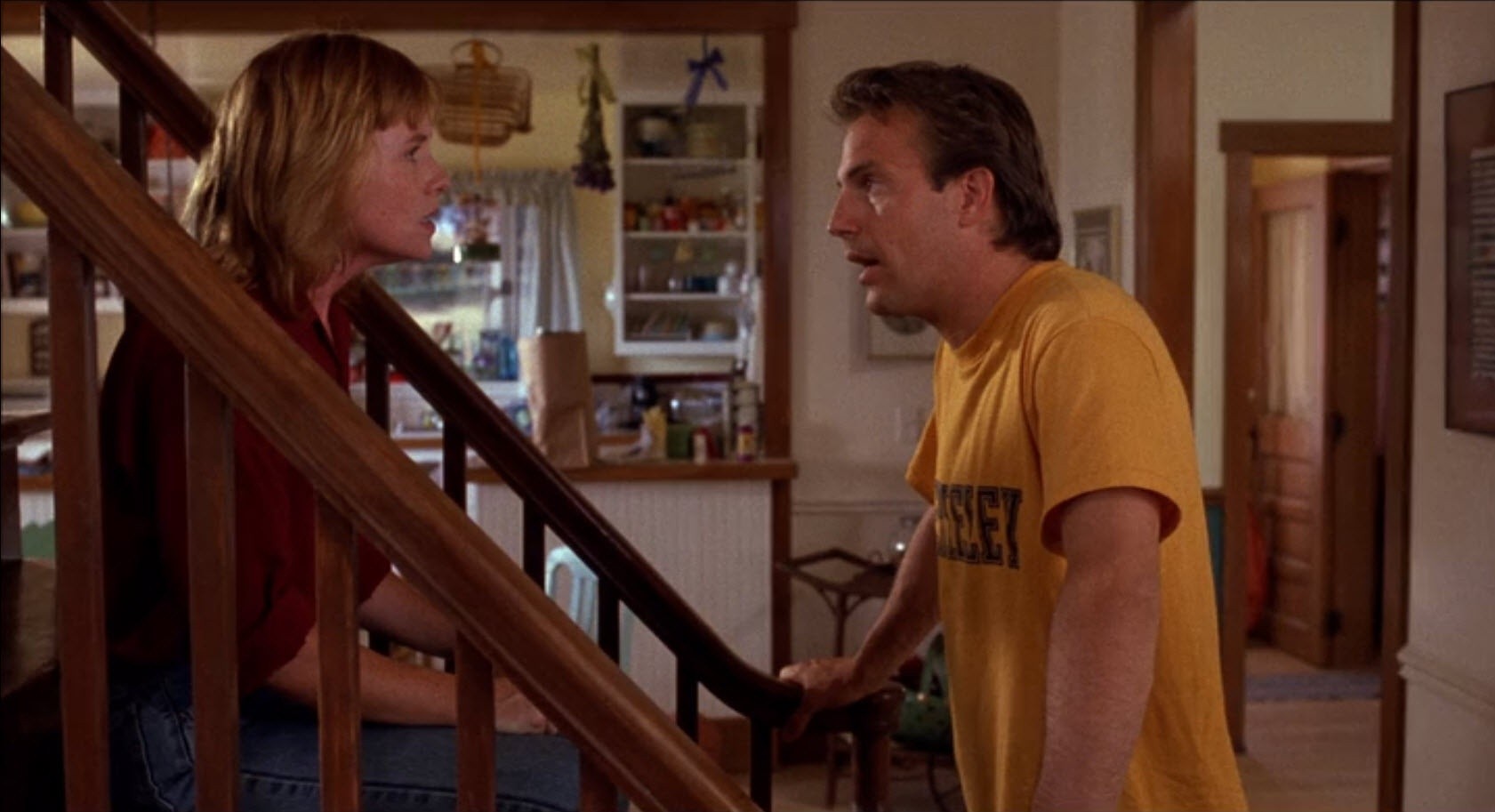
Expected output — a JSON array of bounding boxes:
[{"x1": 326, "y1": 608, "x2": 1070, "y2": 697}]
[
  {"x1": 684, "y1": 121, "x2": 720, "y2": 157},
  {"x1": 593, "y1": 432, "x2": 646, "y2": 463}
]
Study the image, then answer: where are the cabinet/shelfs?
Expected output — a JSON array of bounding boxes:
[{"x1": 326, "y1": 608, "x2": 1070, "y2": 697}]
[
  {"x1": 781, "y1": 547, "x2": 964, "y2": 812},
  {"x1": 0, "y1": 90, "x2": 123, "y2": 315},
  {"x1": 1248, "y1": 167, "x2": 1378, "y2": 670},
  {"x1": 614, "y1": 90, "x2": 762, "y2": 356}
]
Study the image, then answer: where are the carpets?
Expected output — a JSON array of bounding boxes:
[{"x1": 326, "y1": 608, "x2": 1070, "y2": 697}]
[{"x1": 1245, "y1": 670, "x2": 1383, "y2": 702}]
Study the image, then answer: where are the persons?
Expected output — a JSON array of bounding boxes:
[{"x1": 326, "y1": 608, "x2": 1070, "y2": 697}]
[
  {"x1": 779, "y1": 57, "x2": 1245, "y2": 812},
  {"x1": 99, "y1": 30, "x2": 583, "y2": 811}
]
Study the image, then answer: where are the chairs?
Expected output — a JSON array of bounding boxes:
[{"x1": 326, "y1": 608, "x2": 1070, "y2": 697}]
[{"x1": 548, "y1": 544, "x2": 630, "y2": 671}]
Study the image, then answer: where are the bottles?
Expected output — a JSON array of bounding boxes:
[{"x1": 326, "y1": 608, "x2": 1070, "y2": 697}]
[
  {"x1": 888, "y1": 517, "x2": 920, "y2": 569},
  {"x1": 736, "y1": 425, "x2": 754, "y2": 460},
  {"x1": 642, "y1": 406, "x2": 667, "y2": 460},
  {"x1": 695, "y1": 422, "x2": 720, "y2": 460},
  {"x1": 625, "y1": 197, "x2": 746, "y2": 232}
]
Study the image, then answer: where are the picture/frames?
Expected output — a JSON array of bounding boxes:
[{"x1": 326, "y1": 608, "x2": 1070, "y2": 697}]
[
  {"x1": 1071, "y1": 204, "x2": 1123, "y2": 284},
  {"x1": 867, "y1": 310, "x2": 940, "y2": 360}
]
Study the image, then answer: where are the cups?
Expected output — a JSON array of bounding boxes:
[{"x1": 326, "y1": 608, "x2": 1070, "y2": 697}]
[{"x1": 669, "y1": 425, "x2": 693, "y2": 458}]
